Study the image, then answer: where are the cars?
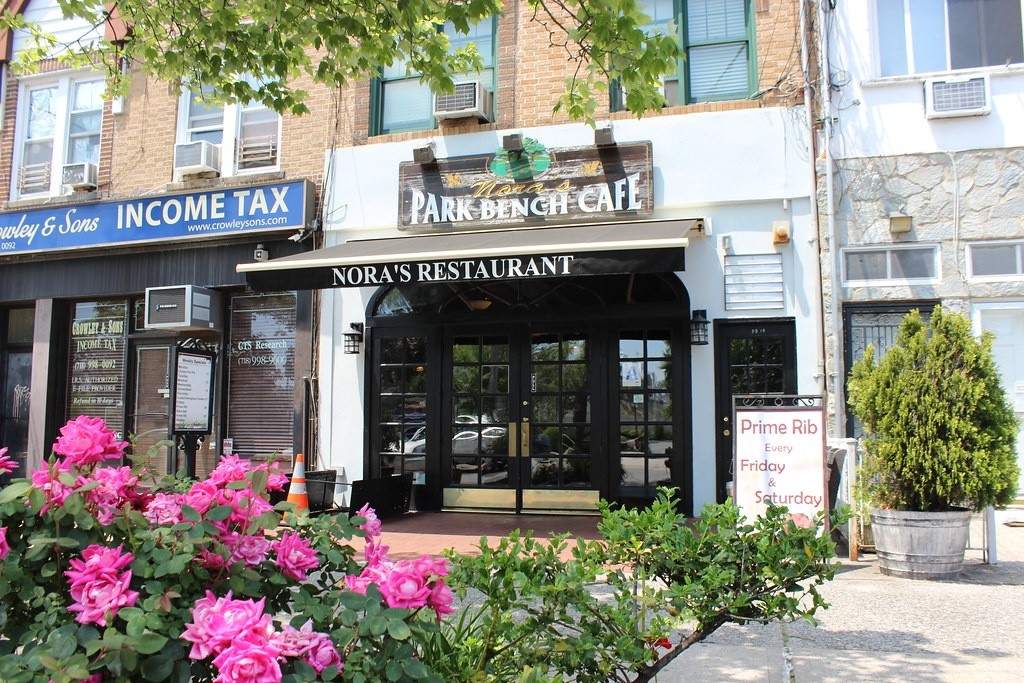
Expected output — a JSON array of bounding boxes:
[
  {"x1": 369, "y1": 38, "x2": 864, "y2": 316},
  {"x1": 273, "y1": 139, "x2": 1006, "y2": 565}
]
[{"x1": 388, "y1": 415, "x2": 507, "y2": 466}]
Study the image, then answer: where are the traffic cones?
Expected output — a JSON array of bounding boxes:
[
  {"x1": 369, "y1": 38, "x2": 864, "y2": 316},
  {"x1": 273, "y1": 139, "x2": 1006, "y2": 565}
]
[{"x1": 279, "y1": 453, "x2": 316, "y2": 527}]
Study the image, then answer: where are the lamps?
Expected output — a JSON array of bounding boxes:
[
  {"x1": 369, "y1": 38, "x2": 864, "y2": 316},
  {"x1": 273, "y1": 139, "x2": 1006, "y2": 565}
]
[
  {"x1": 594, "y1": 123, "x2": 617, "y2": 147},
  {"x1": 413, "y1": 142, "x2": 436, "y2": 164},
  {"x1": 890, "y1": 210, "x2": 914, "y2": 235},
  {"x1": 501, "y1": 133, "x2": 523, "y2": 152},
  {"x1": 689, "y1": 308, "x2": 711, "y2": 346},
  {"x1": 342, "y1": 322, "x2": 364, "y2": 355}
]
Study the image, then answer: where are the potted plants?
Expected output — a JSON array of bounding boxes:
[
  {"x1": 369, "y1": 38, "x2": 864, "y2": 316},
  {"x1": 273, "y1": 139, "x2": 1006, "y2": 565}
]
[{"x1": 847, "y1": 303, "x2": 1021, "y2": 580}]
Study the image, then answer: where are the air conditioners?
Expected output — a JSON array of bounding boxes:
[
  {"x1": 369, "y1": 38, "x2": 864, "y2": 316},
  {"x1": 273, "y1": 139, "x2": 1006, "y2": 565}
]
[
  {"x1": 174, "y1": 139, "x2": 221, "y2": 175},
  {"x1": 433, "y1": 81, "x2": 492, "y2": 121},
  {"x1": 923, "y1": 73, "x2": 993, "y2": 120},
  {"x1": 61, "y1": 163, "x2": 98, "y2": 188},
  {"x1": 144, "y1": 285, "x2": 224, "y2": 333}
]
